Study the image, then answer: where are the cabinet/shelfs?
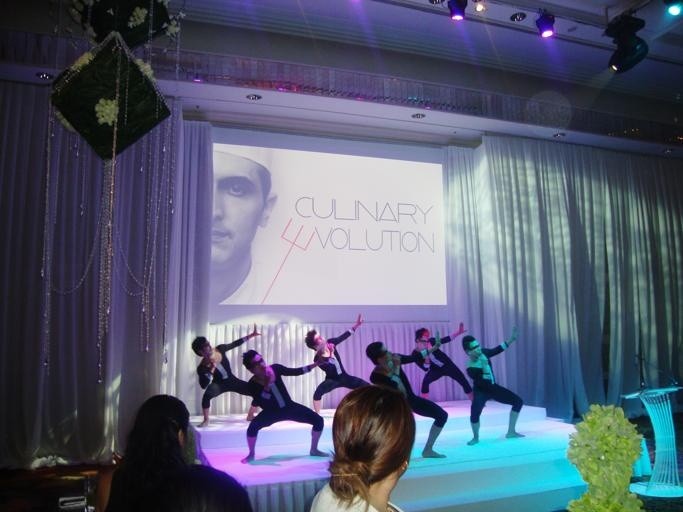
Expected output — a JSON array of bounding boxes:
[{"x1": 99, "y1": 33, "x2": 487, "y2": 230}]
[{"x1": 1, "y1": 464, "x2": 113, "y2": 512}]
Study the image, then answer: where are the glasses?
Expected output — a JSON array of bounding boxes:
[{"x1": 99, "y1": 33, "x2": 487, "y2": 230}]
[
  {"x1": 419, "y1": 340, "x2": 429, "y2": 342},
  {"x1": 253, "y1": 358, "x2": 263, "y2": 364},
  {"x1": 379, "y1": 350, "x2": 387, "y2": 357},
  {"x1": 469, "y1": 344, "x2": 480, "y2": 350},
  {"x1": 204, "y1": 344, "x2": 210, "y2": 347}
]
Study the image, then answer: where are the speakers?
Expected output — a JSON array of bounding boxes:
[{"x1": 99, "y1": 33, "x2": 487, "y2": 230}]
[
  {"x1": 69, "y1": 0, "x2": 170, "y2": 51},
  {"x1": 48, "y1": 36, "x2": 172, "y2": 164}
]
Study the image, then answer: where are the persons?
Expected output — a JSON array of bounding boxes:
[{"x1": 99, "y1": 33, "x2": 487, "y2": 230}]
[
  {"x1": 242, "y1": 350, "x2": 330, "y2": 463},
  {"x1": 210, "y1": 125, "x2": 278, "y2": 308},
  {"x1": 305, "y1": 313, "x2": 371, "y2": 414},
  {"x1": 414, "y1": 321, "x2": 485, "y2": 407},
  {"x1": 139, "y1": 463, "x2": 253, "y2": 512},
  {"x1": 463, "y1": 326, "x2": 526, "y2": 445},
  {"x1": 103, "y1": 394, "x2": 189, "y2": 511},
  {"x1": 192, "y1": 323, "x2": 261, "y2": 427},
  {"x1": 365, "y1": 329, "x2": 448, "y2": 458},
  {"x1": 310, "y1": 385, "x2": 415, "y2": 512}
]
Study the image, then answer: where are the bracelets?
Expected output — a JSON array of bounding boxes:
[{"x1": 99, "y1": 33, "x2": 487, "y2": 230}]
[
  {"x1": 246, "y1": 336, "x2": 248, "y2": 340},
  {"x1": 352, "y1": 327, "x2": 356, "y2": 332}
]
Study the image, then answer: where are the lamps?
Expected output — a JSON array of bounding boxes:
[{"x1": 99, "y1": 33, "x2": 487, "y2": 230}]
[
  {"x1": 536, "y1": 14, "x2": 555, "y2": 38},
  {"x1": 447, "y1": 0, "x2": 468, "y2": 21},
  {"x1": 601, "y1": 14, "x2": 649, "y2": 73}
]
